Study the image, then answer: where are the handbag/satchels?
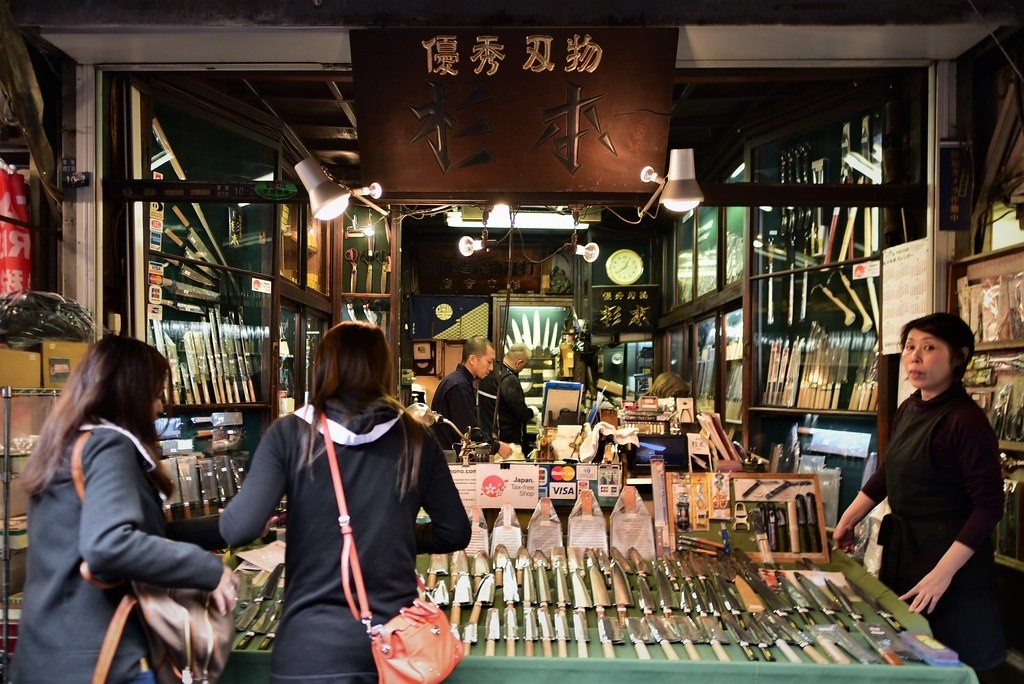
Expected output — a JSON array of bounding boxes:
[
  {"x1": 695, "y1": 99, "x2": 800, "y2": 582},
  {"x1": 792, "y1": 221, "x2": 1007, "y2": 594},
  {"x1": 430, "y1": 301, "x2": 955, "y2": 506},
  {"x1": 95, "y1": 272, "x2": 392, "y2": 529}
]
[{"x1": 360, "y1": 593, "x2": 466, "y2": 683}]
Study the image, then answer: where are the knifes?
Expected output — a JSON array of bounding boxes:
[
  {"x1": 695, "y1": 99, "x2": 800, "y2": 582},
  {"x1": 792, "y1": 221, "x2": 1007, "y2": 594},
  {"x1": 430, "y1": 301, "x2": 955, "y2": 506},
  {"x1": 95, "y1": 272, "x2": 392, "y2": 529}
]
[
  {"x1": 151, "y1": 454, "x2": 236, "y2": 522},
  {"x1": 761, "y1": 321, "x2": 879, "y2": 412},
  {"x1": 234, "y1": 563, "x2": 285, "y2": 651},
  {"x1": 158, "y1": 205, "x2": 223, "y2": 314},
  {"x1": 422, "y1": 544, "x2": 921, "y2": 667}
]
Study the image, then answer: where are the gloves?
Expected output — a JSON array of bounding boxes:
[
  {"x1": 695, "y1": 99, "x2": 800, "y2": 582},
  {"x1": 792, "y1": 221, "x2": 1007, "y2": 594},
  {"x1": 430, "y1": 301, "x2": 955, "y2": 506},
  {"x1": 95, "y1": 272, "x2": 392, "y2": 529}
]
[{"x1": 527, "y1": 403, "x2": 539, "y2": 414}]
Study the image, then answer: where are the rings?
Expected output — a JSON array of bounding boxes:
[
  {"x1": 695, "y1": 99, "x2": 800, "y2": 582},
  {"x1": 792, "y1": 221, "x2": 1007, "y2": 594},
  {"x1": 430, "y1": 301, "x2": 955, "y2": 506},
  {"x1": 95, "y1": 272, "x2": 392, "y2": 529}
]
[{"x1": 230, "y1": 585, "x2": 235, "y2": 592}]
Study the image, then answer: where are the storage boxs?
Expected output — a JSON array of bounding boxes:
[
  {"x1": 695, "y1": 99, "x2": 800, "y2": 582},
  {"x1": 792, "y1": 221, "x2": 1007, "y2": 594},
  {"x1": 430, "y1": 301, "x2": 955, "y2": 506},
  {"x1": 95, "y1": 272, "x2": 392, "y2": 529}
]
[
  {"x1": 0, "y1": 348, "x2": 41, "y2": 388},
  {"x1": 40, "y1": 341, "x2": 91, "y2": 388}
]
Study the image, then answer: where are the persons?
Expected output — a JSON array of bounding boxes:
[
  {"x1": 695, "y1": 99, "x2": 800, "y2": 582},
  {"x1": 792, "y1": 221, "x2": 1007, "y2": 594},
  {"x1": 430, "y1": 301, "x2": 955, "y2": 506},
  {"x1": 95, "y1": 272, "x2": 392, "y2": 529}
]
[
  {"x1": 552, "y1": 269, "x2": 571, "y2": 293},
  {"x1": 643, "y1": 373, "x2": 691, "y2": 398},
  {"x1": 479, "y1": 344, "x2": 539, "y2": 455},
  {"x1": 432, "y1": 336, "x2": 513, "y2": 459},
  {"x1": 8, "y1": 335, "x2": 241, "y2": 684},
  {"x1": 220, "y1": 321, "x2": 472, "y2": 684},
  {"x1": 831, "y1": 312, "x2": 1011, "y2": 684}
]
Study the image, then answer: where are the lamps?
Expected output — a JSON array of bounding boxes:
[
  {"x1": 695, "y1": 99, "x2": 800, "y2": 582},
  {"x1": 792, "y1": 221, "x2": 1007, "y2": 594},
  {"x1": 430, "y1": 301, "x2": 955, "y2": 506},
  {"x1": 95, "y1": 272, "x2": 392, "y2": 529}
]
[
  {"x1": 459, "y1": 229, "x2": 498, "y2": 257},
  {"x1": 352, "y1": 182, "x2": 382, "y2": 199},
  {"x1": 640, "y1": 148, "x2": 705, "y2": 212},
  {"x1": 561, "y1": 232, "x2": 599, "y2": 263},
  {"x1": 294, "y1": 156, "x2": 351, "y2": 220}
]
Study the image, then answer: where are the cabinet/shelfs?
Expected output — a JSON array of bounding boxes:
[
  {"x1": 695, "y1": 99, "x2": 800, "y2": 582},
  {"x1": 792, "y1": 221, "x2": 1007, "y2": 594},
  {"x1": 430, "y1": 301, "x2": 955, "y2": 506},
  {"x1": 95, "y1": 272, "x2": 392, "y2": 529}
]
[
  {"x1": 945, "y1": 241, "x2": 1024, "y2": 573},
  {"x1": 0, "y1": 384, "x2": 59, "y2": 684},
  {"x1": 116, "y1": 72, "x2": 397, "y2": 437},
  {"x1": 660, "y1": 90, "x2": 897, "y2": 469}
]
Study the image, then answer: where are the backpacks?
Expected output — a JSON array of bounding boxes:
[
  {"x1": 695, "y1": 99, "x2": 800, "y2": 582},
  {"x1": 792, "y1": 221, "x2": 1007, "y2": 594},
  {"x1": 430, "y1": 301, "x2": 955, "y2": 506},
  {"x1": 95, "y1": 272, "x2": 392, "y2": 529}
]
[{"x1": 70, "y1": 430, "x2": 236, "y2": 684}]
